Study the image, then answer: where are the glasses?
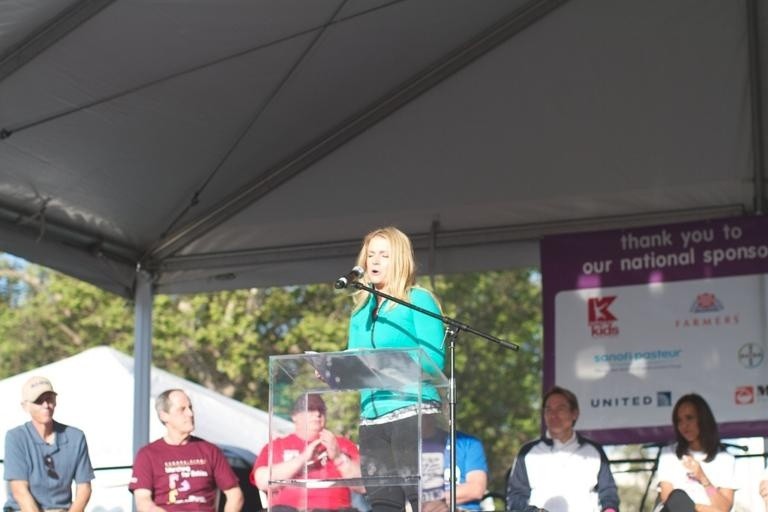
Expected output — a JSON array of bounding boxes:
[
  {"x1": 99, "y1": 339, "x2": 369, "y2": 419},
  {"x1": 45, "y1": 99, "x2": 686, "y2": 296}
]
[{"x1": 45, "y1": 456, "x2": 59, "y2": 480}]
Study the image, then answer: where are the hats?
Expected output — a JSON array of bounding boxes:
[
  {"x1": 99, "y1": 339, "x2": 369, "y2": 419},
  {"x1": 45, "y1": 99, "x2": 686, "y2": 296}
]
[{"x1": 22, "y1": 376, "x2": 58, "y2": 403}]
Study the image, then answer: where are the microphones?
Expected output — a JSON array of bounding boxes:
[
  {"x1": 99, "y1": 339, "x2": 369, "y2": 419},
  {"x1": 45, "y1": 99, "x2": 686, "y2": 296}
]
[{"x1": 335, "y1": 266, "x2": 364, "y2": 289}]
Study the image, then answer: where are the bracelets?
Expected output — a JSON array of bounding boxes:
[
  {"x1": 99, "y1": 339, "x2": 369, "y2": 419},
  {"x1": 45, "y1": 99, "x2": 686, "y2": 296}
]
[{"x1": 703, "y1": 482, "x2": 712, "y2": 488}]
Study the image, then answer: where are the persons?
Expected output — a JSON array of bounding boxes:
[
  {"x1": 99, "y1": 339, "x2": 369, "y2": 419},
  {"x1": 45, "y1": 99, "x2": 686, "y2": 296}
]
[
  {"x1": 760, "y1": 465, "x2": 768, "y2": 511},
  {"x1": 444, "y1": 431, "x2": 487, "y2": 511},
  {"x1": 418, "y1": 413, "x2": 446, "y2": 512},
  {"x1": 651, "y1": 391, "x2": 741, "y2": 512},
  {"x1": 128, "y1": 387, "x2": 244, "y2": 512},
  {"x1": 3, "y1": 375, "x2": 97, "y2": 511},
  {"x1": 312, "y1": 224, "x2": 449, "y2": 511},
  {"x1": 502, "y1": 386, "x2": 621, "y2": 512},
  {"x1": 248, "y1": 392, "x2": 367, "y2": 510}
]
